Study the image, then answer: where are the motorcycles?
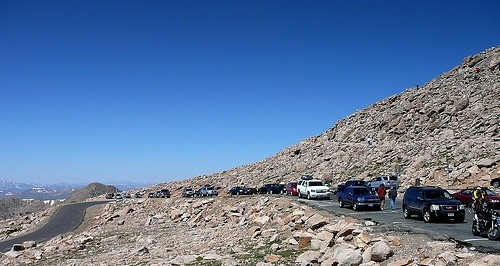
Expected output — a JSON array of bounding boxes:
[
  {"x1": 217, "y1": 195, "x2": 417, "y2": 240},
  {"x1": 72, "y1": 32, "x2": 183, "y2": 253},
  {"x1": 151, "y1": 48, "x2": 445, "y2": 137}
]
[{"x1": 467, "y1": 199, "x2": 500, "y2": 241}]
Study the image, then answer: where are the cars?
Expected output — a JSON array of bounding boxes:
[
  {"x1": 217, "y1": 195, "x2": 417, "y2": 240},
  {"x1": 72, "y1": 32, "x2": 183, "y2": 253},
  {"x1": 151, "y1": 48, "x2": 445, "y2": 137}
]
[
  {"x1": 336, "y1": 180, "x2": 385, "y2": 211},
  {"x1": 123, "y1": 192, "x2": 131, "y2": 198},
  {"x1": 228, "y1": 180, "x2": 298, "y2": 196},
  {"x1": 452, "y1": 186, "x2": 500, "y2": 211},
  {"x1": 147, "y1": 189, "x2": 171, "y2": 198},
  {"x1": 105, "y1": 193, "x2": 113, "y2": 199},
  {"x1": 115, "y1": 193, "x2": 122, "y2": 199},
  {"x1": 181, "y1": 183, "x2": 218, "y2": 197},
  {"x1": 135, "y1": 191, "x2": 144, "y2": 198}
]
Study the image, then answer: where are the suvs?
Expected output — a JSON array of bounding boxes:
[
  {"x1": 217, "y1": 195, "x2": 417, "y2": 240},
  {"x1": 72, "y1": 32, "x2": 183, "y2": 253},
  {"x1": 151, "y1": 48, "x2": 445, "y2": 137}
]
[
  {"x1": 296, "y1": 174, "x2": 331, "y2": 200},
  {"x1": 366, "y1": 174, "x2": 401, "y2": 191},
  {"x1": 402, "y1": 186, "x2": 466, "y2": 223}
]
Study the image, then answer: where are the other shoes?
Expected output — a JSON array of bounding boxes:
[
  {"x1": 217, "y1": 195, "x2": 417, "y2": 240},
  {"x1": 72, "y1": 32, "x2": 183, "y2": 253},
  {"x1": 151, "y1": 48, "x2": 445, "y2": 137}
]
[{"x1": 381, "y1": 208, "x2": 383, "y2": 210}]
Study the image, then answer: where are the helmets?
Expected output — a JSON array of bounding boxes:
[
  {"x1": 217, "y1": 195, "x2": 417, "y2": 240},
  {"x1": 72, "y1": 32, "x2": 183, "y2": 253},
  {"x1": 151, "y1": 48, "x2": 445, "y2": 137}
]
[{"x1": 476, "y1": 187, "x2": 481, "y2": 190}]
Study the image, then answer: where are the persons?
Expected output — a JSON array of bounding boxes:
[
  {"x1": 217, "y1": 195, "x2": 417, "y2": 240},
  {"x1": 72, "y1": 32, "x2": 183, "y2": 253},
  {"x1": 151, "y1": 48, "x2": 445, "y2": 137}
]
[
  {"x1": 378, "y1": 183, "x2": 387, "y2": 208},
  {"x1": 388, "y1": 186, "x2": 398, "y2": 210},
  {"x1": 472, "y1": 187, "x2": 484, "y2": 208}
]
